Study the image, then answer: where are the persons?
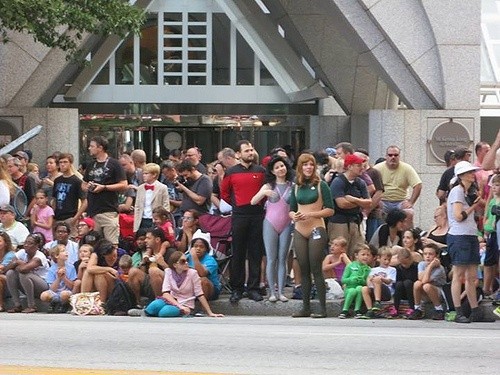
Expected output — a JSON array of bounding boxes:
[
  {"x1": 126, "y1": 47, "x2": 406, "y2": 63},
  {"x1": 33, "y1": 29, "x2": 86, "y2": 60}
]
[{"x1": 0, "y1": 131, "x2": 500, "y2": 323}]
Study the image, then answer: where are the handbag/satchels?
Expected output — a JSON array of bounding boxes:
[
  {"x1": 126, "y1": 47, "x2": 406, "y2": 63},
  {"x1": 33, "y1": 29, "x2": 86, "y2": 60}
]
[
  {"x1": 69, "y1": 291, "x2": 105, "y2": 316},
  {"x1": 105, "y1": 278, "x2": 137, "y2": 315}
]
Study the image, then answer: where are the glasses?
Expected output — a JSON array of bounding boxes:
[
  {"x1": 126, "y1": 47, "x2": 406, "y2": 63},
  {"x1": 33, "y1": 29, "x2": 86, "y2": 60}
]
[
  {"x1": 76, "y1": 224, "x2": 87, "y2": 227},
  {"x1": 181, "y1": 217, "x2": 195, "y2": 221},
  {"x1": 176, "y1": 257, "x2": 190, "y2": 265},
  {"x1": 387, "y1": 154, "x2": 399, "y2": 157}
]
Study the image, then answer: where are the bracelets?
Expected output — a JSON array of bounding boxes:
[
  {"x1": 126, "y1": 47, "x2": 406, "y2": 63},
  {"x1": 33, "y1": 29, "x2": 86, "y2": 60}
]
[{"x1": 104, "y1": 184, "x2": 107, "y2": 190}]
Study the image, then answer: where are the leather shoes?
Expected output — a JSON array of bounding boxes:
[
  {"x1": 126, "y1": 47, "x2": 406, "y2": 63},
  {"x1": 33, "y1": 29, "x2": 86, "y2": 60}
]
[
  {"x1": 229, "y1": 289, "x2": 244, "y2": 303},
  {"x1": 246, "y1": 290, "x2": 264, "y2": 302}
]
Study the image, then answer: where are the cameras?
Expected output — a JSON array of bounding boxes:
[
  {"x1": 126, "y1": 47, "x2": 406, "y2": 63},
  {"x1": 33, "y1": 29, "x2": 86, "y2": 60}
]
[{"x1": 173, "y1": 175, "x2": 190, "y2": 193}]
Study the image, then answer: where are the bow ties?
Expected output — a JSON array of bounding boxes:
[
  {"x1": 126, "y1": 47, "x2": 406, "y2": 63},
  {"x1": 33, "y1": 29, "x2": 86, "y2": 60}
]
[{"x1": 144, "y1": 185, "x2": 154, "y2": 190}]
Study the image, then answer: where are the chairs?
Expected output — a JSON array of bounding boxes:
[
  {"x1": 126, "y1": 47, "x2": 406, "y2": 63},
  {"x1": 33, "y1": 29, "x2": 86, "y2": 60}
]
[{"x1": 198, "y1": 213, "x2": 232, "y2": 293}]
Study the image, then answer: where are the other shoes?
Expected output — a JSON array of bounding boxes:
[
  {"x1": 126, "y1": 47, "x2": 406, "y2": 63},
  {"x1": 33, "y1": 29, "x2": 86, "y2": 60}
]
[
  {"x1": 128, "y1": 306, "x2": 147, "y2": 316},
  {"x1": 337, "y1": 288, "x2": 500, "y2": 324}
]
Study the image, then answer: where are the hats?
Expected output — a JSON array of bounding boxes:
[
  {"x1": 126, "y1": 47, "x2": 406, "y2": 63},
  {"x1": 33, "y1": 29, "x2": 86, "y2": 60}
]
[
  {"x1": 12, "y1": 151, "x2": 28, "y2": 160},
  {"x1": 449, "y1": 161, "x2": 479, "y2": 185},
  {"x1": 79, "y1": 217, "x2": 95, "y2": 231},
  {"x1": 444, "y1": 150, "x2": 457, "y2": 168},
  {"x1": 0, "y1": 204, "x2": 16, "y2": 213},
  {"x1": 344, "y1": 154, "x2": 366, "y2": 166},
  {"x1": 325, "y1": 148, "x2": 337, "y2": 156}
]
